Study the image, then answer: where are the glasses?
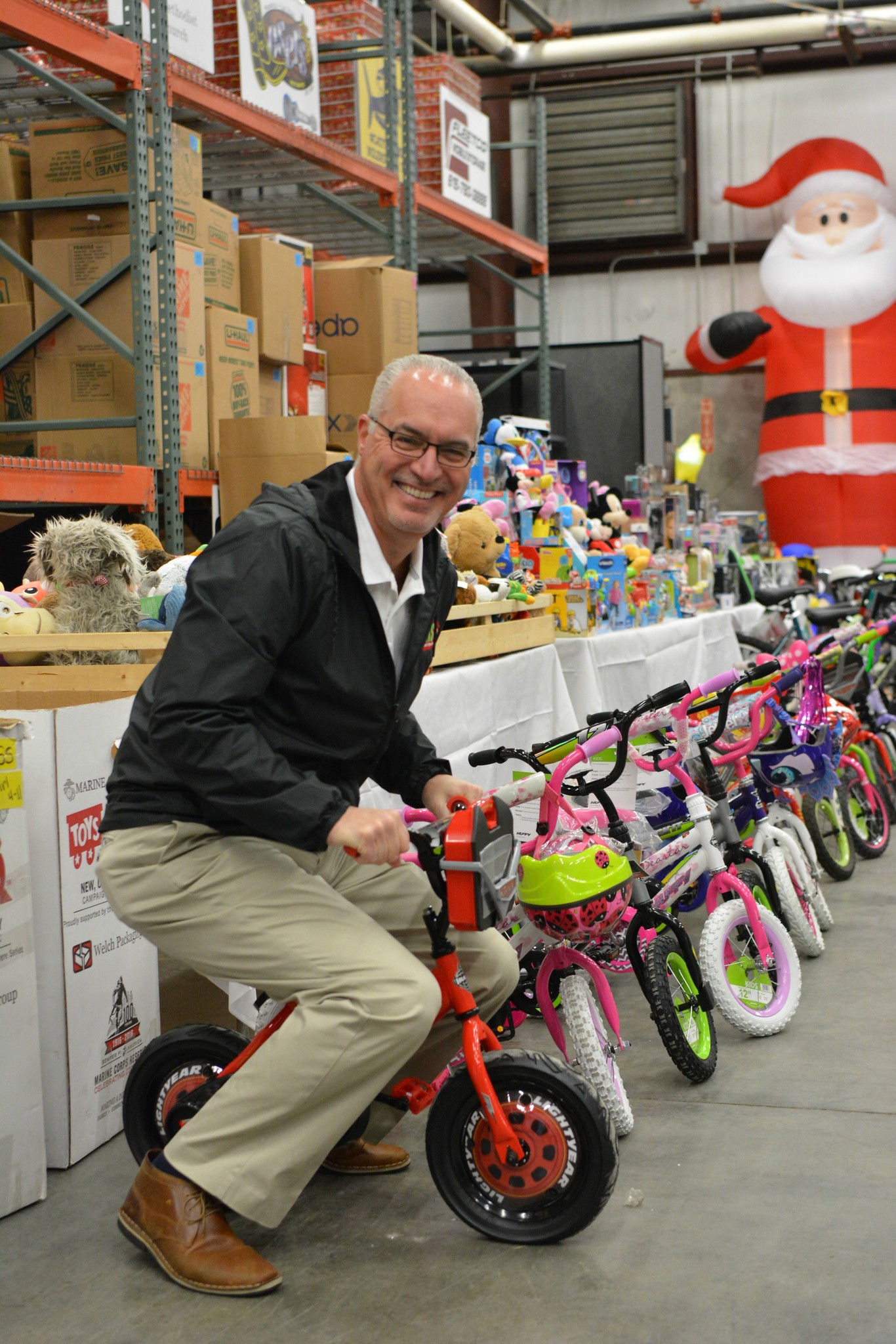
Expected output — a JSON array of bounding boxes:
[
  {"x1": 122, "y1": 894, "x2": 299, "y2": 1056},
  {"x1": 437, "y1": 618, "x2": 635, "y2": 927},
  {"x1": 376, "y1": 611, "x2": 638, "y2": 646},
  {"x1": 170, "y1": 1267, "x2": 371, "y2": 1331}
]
[{"x1": 370, "y1": 418, "x2": 477, "y2": 468}]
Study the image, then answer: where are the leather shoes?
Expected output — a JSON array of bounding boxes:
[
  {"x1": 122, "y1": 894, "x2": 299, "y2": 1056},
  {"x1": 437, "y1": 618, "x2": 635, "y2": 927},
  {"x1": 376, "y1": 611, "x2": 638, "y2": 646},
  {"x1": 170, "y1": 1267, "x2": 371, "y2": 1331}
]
[
  {"x1": 323, "y1": 1139, "x2": 412, "y2": 1174},
  {"x1": 115, "y1": 1148, "x2": 282, "y2": 1296}
]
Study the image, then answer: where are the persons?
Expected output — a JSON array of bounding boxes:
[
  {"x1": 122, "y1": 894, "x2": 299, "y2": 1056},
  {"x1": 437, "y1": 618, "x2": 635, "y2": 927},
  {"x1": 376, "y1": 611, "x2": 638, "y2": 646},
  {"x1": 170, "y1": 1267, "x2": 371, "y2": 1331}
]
[
  {"x1": 98, "y1": 353, "x2": 521, "y2": 1296},
  {"x1": 684, "y1": 136, "x2": 896, "y2": 584}
]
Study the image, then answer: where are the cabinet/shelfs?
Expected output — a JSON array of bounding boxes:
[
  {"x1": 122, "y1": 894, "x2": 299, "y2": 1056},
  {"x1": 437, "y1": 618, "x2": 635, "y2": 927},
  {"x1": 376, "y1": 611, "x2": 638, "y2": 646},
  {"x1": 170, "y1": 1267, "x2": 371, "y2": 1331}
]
[{"x1": 0, "y1": 0, "x2": 553, "y2": 549}]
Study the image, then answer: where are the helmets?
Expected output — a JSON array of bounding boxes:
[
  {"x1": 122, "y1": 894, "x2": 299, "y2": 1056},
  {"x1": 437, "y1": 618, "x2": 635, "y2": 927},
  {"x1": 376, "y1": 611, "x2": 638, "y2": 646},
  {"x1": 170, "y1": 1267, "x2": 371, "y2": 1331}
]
[{"x1": 515, "y1": 844, "x2": 633, "y2": 944}]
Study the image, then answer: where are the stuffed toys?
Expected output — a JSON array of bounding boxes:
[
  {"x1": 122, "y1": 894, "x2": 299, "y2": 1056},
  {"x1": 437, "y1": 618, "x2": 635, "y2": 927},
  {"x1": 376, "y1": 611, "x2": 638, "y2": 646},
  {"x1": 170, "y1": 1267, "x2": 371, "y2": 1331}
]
[
  {"x1": 430, "y1": 416, "x2": 655, "y2": 658},
  {"x1": 0, "y1": 517, "x2": 215, "y2": 667}
]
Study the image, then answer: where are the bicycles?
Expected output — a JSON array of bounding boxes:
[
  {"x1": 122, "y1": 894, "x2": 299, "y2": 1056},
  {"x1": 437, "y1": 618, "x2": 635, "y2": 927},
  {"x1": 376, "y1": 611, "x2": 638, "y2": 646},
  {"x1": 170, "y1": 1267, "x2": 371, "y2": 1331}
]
[{"x1": 121, "y1": 545, "x2": 896, "y2": 1245}]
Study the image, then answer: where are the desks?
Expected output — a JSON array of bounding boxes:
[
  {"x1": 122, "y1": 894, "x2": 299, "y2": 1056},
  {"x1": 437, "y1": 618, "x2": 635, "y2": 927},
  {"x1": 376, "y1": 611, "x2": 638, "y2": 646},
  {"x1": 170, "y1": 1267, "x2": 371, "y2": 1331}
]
[{"x1": 0, "y1": 598, "x2": 767, "y2": 1216}]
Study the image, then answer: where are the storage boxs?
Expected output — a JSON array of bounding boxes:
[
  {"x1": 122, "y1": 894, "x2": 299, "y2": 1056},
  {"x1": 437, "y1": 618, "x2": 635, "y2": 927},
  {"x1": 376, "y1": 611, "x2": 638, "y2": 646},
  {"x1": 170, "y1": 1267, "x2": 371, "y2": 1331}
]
[
  {"x1": 0, "y1": 114, "x2": 419, "y2": 549},
  {"x1": 474, "y1": 416, "x2": 685, "y2": 628}
]
